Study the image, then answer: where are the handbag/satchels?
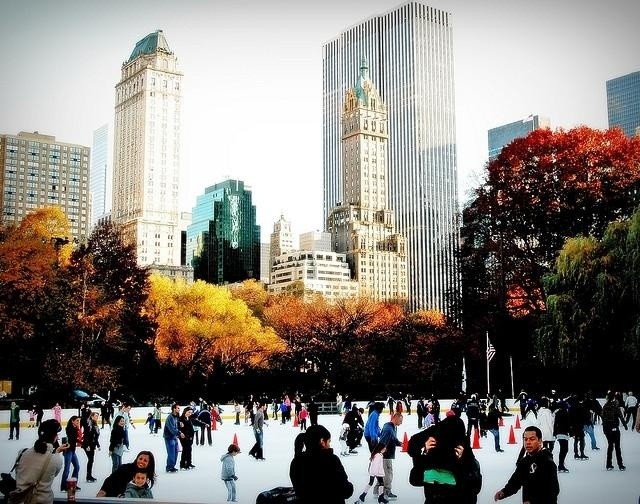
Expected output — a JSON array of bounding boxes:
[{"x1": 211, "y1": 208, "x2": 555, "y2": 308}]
[{"x1": 0, "y1": 473, "x2": 39, "y2": 504}]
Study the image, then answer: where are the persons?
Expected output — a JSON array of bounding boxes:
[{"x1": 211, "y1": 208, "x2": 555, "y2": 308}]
[{"x1": 1, "y1": 390, "x2": 640, "y2": 502}]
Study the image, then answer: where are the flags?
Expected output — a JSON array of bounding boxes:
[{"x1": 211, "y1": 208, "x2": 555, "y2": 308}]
[
  {"x1": 461, "y1": 360, "x2": 466, "y2": 391},
  {"x1": 486, "y1": 333, "x2": 496, "y2": 361}
]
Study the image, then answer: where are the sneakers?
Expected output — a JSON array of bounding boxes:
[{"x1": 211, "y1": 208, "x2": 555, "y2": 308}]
[
  {"x1": 61, "y1": 475, "x2": 97, "y2": 491},
  {"x1": 558, "y1": 448, "x2": 625, "y2": 470},
  {"x1": 167, "y1": 464, "x2": 195, "y2": 472},
  {"x1": 355, "y1": 493, "x2": 397, "y2": 504}
]
